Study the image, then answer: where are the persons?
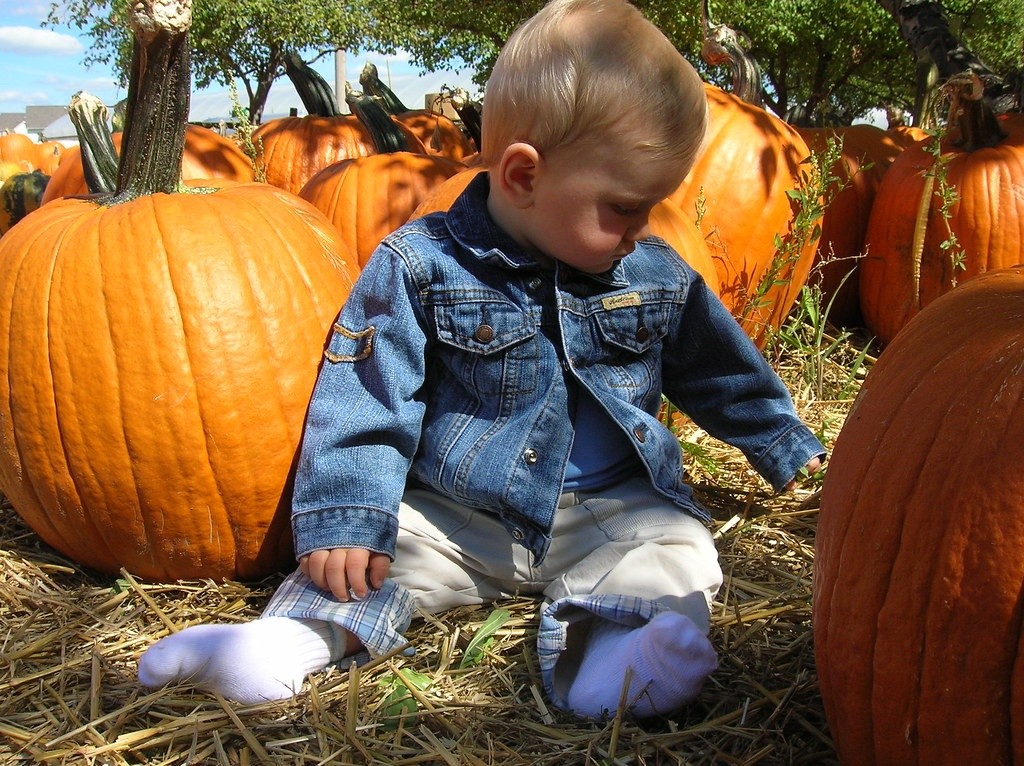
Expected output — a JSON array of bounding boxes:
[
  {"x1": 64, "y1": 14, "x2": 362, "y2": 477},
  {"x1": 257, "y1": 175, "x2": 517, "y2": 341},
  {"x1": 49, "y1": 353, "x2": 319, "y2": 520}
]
[{"x1": 137, "y1": 0, "x2": 828, "y2": 723}]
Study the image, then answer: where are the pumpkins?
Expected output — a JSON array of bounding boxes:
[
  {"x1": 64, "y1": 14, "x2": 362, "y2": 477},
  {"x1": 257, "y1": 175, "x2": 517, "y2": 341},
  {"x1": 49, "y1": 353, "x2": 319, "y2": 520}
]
[
  {"x1": 0, "y1": 0, "x2": 1024, "y2": 585},
  {"x1": 809, "y1": 261, "x2": 1024, "y2": 766}
]
